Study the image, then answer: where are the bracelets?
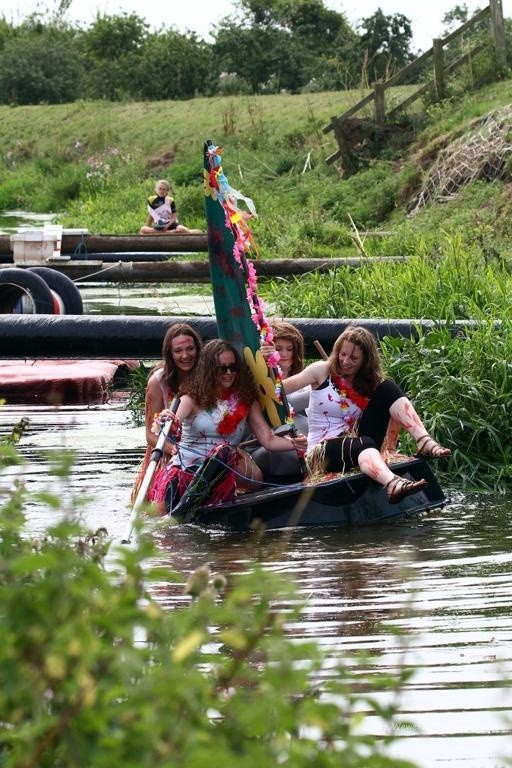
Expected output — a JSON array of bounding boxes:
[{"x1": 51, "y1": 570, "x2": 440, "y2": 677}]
[
  {"x1": 152, "y1": 413, "x2": 159, "y2": 433},
  {"x1": 169, "y1": 425, "x2": 187, "y2": 440}
]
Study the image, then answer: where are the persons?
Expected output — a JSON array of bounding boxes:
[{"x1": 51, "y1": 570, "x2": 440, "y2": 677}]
[
  {"x1": 259, "y1": 325, "x2": 451, "y2": 503},
  {"x1": 142, "y1": 179, "x2": 204, "y2": 235},
  {"x1": 269, "y1": 322, "x2": 310, "y2": 435},
  {"x1": 158, "y1": 339, "x2": 308, "y2": 523},
  {"x1": 145, "y1": 322, "x2": 201, "y2": 456}
]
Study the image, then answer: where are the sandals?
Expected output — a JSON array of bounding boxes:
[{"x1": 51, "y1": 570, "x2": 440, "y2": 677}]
[
  {"x1": 413, "y1": 434, "x2": 451, "y2": 458},
  {"x1": 383, "y1": 475, "x2": 428, "y2": 505}
]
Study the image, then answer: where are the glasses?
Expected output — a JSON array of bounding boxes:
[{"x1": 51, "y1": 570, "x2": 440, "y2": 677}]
[{"x1": 215, "y1": 364, "x2": 238, "y2": 376}]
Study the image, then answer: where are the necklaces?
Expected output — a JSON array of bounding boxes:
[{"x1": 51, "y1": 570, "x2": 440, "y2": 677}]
[
  {"x1": 211, "y1": 389, "x2": 251, "y2": 434},
  {"x1": 332, "y1": 374, "x2": 368, "y2": 409},
  {"x1": 340, "y1": 378, "x2": 355, "y2": 427}
]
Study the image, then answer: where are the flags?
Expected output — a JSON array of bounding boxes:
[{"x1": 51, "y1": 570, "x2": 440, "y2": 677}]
[{"x1": 202, "y1": 138, "x2": 293, "y2": 436}]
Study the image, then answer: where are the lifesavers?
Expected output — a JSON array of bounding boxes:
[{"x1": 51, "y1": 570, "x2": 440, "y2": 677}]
[
  {"x1": 0, "y1": 266, "x2": 54, "y2": 315},
  {"x1": 24, "y1": 266, "x2": 85, "y2": 314}
]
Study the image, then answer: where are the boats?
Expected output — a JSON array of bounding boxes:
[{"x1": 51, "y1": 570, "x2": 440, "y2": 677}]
[{"x1": 175, "y1": 453, "x2": 453, "y2": 530}]
